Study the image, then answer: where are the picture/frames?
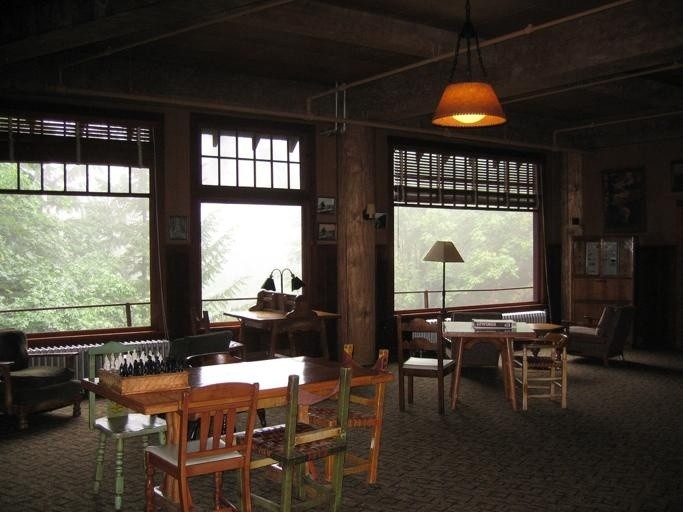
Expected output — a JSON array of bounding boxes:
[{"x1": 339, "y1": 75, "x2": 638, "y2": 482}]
[
  {"x1": 314, "y1": 196, "x2": 336, "y2": 215},
  {"x1": 168, "y1": 215, "x2": 189, "y2": 241},
  {"x1": 317, "y1": 223, "x2": 338, "y2": 241},
  {"x1": 374, "y1": 213, "x2": 389, "y2": 231}
]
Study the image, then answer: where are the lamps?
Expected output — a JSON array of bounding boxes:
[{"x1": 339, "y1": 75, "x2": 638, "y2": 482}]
[
  {"x1": 363, "y1": 203, "x2": 376, "y2": 218},
  {"x1": 431, "y1": 0, "x2": 508, "y2": 127},
  {"x1": 261, "y1": 268, "x2": 306, "y2": 294},
  {"x1": 422, "y1": 240, "x2": 464, "y2": 320}
]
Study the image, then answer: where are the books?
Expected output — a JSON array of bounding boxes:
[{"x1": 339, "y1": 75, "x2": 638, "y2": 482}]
[{"x1": 472, "y1": 319, "x2": 515, "y2": 331}]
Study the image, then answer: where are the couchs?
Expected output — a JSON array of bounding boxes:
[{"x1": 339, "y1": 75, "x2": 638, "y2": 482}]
[
  {"x1": 168, "y1": 330, "x2": 234, "y2": 370},
  {"x1": 560, "y1": 303, "x2": 637, "y2": 368},
  {"x1": 442, "y1": 311, "x2": 502, "y2": 369},
  {"x1": 0, "y1": 328, "x2": 85, "y2": 431}
]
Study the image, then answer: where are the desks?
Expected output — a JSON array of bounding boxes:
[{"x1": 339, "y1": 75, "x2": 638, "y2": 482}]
[
  {"x1": 81, "y1": 355, "x2": 395, "y2": 512},
  {"x1": 223, "y1": 308, "x2": 342, "y2": 362},
  {"x1": 442, "y1": 322, "x2": 536, "y2": 412},
  {"x1": 527, "y1": 322, "x2": 564, "y2": 358}
]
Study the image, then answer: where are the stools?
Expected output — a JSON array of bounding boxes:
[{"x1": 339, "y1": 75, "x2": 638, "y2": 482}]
[{"x1": 92, "y1": 413, "x2": 168, "y2": 512}]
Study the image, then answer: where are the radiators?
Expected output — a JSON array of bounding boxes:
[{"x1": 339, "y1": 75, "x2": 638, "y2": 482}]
[
  {"x1": 24, "y1": 339, "x2": 171, "y2": 395},
  {"x1": 411, "y1": 310, "x2": 546, "y2": 354}
]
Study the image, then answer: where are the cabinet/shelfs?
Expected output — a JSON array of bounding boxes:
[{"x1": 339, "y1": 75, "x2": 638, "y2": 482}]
[{"x1": 560, "y1": 233, "x2": 641, "y2": 327}]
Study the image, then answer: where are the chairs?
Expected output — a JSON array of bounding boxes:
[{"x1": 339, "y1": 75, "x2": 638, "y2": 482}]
[
  {"x1": 512, "y1": 344, "x2": 567, "y2": 412},
  {"x1": 308, "y1": 344, "x2": 389, "y2": 484},
  {"x1": 183, "y1": 352, "x2": 267, "y2": 441},
  {"x1": 144, "y1": 382, "x2": 259, "y2": 512},
  {"x1": 229, "y1": 340, "x2": 247, "y2": 362},
  {"x1": 396, "y1": 316, "x2": 456, "y2": 417},
  {"x1": 220, "y1": 366, "x2": 353, "y2": 512}
]
